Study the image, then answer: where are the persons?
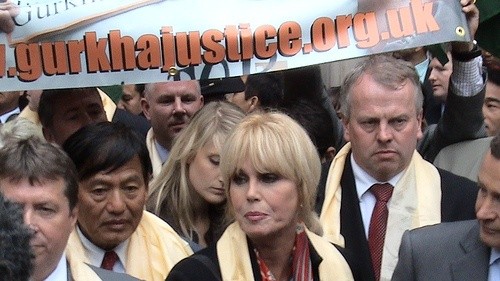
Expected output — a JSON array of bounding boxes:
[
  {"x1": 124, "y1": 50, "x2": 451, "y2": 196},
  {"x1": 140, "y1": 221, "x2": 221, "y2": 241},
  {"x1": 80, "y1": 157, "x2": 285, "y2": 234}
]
[{"x1": 0, "y1": 0, "x2": 500, "y2": 281}]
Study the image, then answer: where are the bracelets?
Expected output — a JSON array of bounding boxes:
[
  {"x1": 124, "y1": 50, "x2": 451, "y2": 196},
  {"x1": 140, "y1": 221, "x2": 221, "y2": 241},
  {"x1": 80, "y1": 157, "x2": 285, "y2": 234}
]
[{"x1": 451, "y1": 40, "x2": 482, "y2": 62}]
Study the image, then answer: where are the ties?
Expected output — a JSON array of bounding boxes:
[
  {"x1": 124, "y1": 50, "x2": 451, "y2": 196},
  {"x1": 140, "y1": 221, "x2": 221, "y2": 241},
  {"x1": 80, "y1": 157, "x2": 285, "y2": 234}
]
[
  {"x1": 367, "y1": 183, "x2": 393, "y2": 281},
  {"x1": 101, "y1": 251, "x2": 119, "y2": 271}
]
